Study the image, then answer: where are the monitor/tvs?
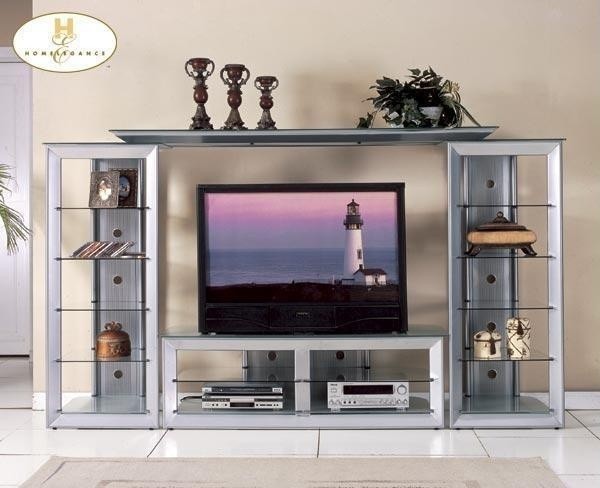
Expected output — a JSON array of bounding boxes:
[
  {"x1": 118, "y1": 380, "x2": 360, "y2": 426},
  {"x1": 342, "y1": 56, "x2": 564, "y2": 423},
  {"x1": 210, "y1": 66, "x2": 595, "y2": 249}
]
[{"x1": 196, "y1": 182, "x2": 408, "y2": 336}]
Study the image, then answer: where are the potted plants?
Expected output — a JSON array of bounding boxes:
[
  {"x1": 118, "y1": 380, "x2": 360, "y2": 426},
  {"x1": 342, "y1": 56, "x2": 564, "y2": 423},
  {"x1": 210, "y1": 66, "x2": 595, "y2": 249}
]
[{"x1": 354, "y1": 66, "x2": 479, "y2": 128}]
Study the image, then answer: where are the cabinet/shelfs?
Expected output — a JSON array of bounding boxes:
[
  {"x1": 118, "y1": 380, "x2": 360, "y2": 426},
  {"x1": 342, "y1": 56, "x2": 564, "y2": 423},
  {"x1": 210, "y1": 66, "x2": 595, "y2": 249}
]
[
  {"x1": 160, "y1": 334, "x2": 445, "y2": 431},
  {"x1": 39, "y1": 141, "x2": 159, "y2": 430},
  {"x1": 448, "y1": 138, "x2": 567, "y2": 430}
]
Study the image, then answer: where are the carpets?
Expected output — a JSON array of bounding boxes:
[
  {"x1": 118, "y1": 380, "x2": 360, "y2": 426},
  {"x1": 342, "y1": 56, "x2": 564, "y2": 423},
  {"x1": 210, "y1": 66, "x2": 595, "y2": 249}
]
[{"x1": 17, "y1": 455, "x2": 568, "y2": 488}]
